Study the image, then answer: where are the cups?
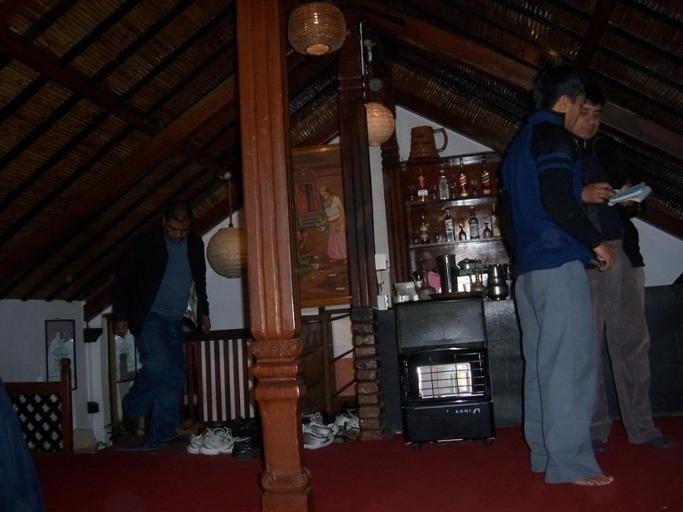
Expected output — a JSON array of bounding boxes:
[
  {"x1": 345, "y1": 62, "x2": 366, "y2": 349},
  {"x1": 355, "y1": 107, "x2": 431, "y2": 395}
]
[
  {"x1": 408, "y1": 124, "x2": 447, "y2": 159},
  {"x1": 436, "y1": 254, "x2": 459, "y2": 294}
]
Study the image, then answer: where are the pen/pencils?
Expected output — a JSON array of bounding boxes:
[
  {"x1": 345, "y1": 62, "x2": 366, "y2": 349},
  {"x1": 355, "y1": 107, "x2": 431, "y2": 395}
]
[{"x1": 584, "y1": 180, "x2": 618, "y2": 194}]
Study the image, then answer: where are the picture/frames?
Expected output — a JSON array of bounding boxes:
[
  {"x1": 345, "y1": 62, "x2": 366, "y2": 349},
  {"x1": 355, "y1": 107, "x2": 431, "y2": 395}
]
[{"x1": 45, "y1": 319, "x2": 76, "y2": 391}]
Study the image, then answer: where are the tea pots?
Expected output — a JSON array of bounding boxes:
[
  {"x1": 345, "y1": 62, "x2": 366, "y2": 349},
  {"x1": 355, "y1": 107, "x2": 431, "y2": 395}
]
[
  {"x1": 410, "y1": 270, "x2": 436, "y2": 300},
  {"x1": 468, "y1": 265, "x2": 485, "y2": 294}
]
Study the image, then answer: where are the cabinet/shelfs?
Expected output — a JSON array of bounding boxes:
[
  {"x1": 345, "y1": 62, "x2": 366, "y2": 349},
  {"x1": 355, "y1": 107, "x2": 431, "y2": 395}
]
[{"x1": 400, "y1": 152, "x2": 511, "y2": 280}]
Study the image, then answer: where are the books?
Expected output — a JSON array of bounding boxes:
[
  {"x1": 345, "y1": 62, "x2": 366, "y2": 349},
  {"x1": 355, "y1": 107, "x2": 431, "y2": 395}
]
[{"x1": 608, "y1": 182, "x2": 651, "y2": 206}]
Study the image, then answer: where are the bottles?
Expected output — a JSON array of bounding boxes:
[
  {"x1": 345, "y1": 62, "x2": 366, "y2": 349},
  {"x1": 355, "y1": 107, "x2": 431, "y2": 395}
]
[
  {"x1": 408, "y1": 159, "x2": 501, "y2": 242},
  {"x1": 488, "y1": 265, "x2": 513, "y2": 301}
]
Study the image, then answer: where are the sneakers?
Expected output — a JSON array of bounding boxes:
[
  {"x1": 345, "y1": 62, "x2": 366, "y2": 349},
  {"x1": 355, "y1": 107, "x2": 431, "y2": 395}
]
[{"x1": 187, "y1": 410, "x2": 360, "y2": 458}]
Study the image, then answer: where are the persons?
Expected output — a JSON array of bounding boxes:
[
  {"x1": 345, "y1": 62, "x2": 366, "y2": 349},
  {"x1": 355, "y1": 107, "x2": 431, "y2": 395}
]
[
  {"x1": 548, "y1": 68, "x2": 673, "y2": 454},
  {"x1": 493, "y1": 67, "x2": 619, "y2": 490},
  {"x1": 109, "y1": 199, "x2": 214, "y2": 444},
  {"x1": 314, "y1": 184, "x2": 348, "y2": 266}
]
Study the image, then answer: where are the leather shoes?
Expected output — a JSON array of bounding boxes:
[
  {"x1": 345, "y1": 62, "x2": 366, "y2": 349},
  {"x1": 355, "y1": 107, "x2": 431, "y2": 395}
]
[
  {"x1": 649, "y1": 437, "x2": 671, "y2": 448},
  {"x1": 593, "y1": 442, "x2": 606, "y2": 452}
]
